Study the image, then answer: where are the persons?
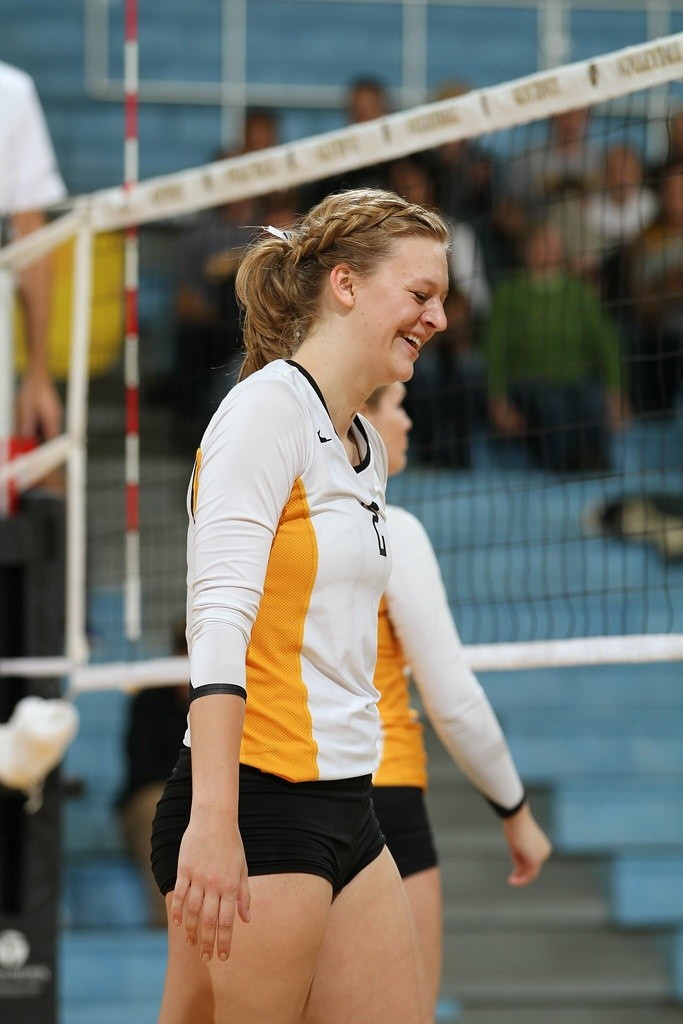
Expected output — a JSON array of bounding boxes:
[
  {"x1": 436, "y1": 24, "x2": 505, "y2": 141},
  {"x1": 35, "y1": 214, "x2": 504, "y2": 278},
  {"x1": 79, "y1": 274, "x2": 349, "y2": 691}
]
[
  {"x1": 357, "y1": 382, "x2": 553, "y2": 1005},
  {"x1": 119, "y1": 623, "x2": 191, "y2": 929},
  {"x1": 588, "y1": 145, "x2": 662, "y2": 239},
  {"x1": 484, "y1": 220, "x2": 615, "y2": 476},
  {"x1": 149, "y1": 188, "x2": 451, "y2": 1023},
  {"x1": 169, "y1": 72, "x2": 681, "y2": 468},
  {"x1": 626, "y1": 174, "x2": 683, "y2": 418},
  {"x1": 0, "y1": 61, "x2": 104, "y2": 515}
]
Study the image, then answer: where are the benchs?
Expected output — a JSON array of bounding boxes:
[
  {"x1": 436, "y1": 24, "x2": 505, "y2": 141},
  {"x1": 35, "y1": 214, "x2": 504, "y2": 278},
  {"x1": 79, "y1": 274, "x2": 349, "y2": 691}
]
[{"x1": 49, "y1": 414, "x2": 683, "y2": 1024}]
